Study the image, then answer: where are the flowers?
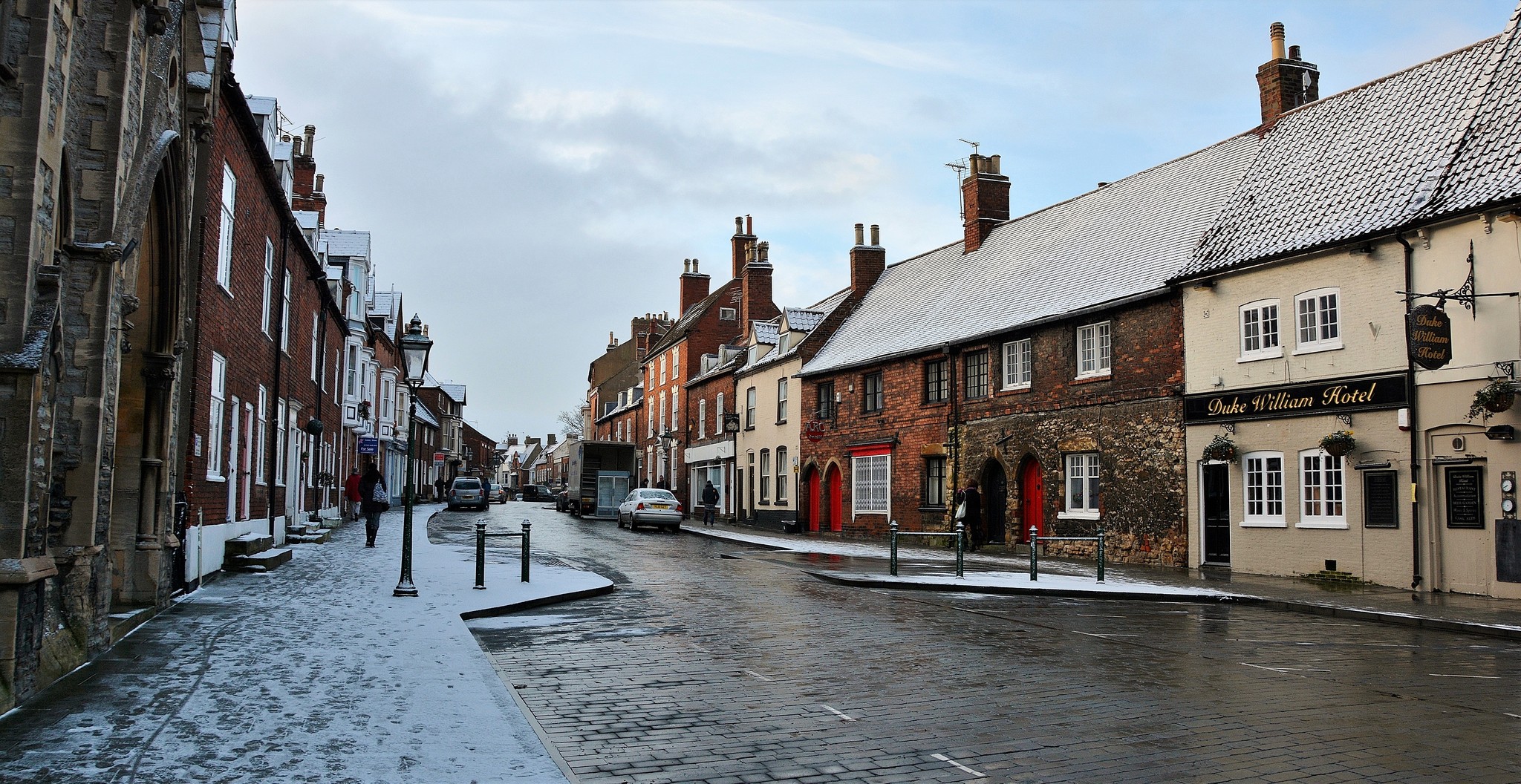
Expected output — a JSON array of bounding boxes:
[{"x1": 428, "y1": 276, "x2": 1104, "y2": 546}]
[
  {"x1": 359, "y1": 399, "x2": 372, "y2": 422},
  {"x1": 1198, "y1": 434, "x2": 1239, "y2": 470},
  {"x1": 1315, "y1": 430, "x2": 1358, "y2": 465}
]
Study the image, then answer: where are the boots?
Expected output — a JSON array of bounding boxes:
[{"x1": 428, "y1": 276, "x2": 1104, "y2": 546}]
[
  {"x1": 365, "y1": 525, "x2": 378, "y2": 548},
  {"x1": 970, "y1": 540, "x2": 976, "y2": 552},
  {"x1": 963, "y1": 538, "x2": 970, "y2": 551}
]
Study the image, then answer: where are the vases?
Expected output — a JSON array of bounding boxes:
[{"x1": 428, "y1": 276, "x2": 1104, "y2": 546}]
[
  {"x1": 359, "y1": 405, "x2": 364, "y2": 411},
  {"x1": 1210, "y1": 449, "x2": 1229, "y2": 461},
  {"x1": 1325, "y1": 441, "x2": 1353, "y2": 458}
]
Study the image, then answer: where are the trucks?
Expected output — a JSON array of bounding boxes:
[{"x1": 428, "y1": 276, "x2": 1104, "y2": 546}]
[{"x1": 567, "y1": 439, "x2": 637, "y2": 518}]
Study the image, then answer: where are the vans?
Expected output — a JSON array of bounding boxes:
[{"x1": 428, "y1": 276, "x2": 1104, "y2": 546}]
[{"x1": 522, "y1": 484, "x2": 556, "y2": 503}]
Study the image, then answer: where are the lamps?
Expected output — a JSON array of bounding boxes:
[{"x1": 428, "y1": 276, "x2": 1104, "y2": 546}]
[{"x1": 1484, "y1": 423, "x2": 1516, "y2": 440}]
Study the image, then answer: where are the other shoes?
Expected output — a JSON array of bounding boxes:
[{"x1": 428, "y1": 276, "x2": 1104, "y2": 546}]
[
  {"x1": 350, "y1": 518, "x2": 354, "y2": 521},
  {"x1": 702, "y1": 521, "x2": 707, "y2": 526},
  {"x1": 355, "y1": 513, "x2": 358, "y2": 522},
  {"x1": 711, "y1": 525, "x2": 713, "y2": 527}
]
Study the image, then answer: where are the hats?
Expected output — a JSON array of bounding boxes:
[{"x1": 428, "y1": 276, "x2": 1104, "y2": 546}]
[{"x1": 644, "y1": 478, "x2": 649, "y2": 482}]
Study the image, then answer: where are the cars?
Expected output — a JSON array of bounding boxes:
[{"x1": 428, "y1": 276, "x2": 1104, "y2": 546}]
[
  {"x1": 488, "y1": 484, "x2": 508, "y2": 504},
  {"x1": 556, "y1": 483, "x2": 569, "y2": 512},
  {"x1": 514, "y1": 494, "x2": 523, "y2": 501},
  {"x1": 618, "y1": 488, "x2": 684, "y2": 534},
  {"x1": 447, "y1": 476, "x2": 485, "y2": 512}
]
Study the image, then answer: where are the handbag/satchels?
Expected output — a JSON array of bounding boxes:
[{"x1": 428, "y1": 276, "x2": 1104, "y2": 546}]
[
  {"x1": 955, "y1": 500, "x2": 966, "y2": 519},
  {"x1": 704, "y1": 503, "x2": 715, "y2": 512},
  {"x1": 381, "y1": 501, "x2": 390, "y2": 512},
  {"x1": 372, "y1": 482, "x2": 388, "y2": 503}
]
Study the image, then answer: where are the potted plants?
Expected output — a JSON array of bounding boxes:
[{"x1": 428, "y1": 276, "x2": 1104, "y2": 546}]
[
  {"x1": 392, "y1": 430, "x2": 400, "y2": 438},
  {"x1": 1462, "y1": 380, "x2": 1517, "y2": 424},
  {"x1": 307, "y1": 510, "x2": 324, "y2": 529}
]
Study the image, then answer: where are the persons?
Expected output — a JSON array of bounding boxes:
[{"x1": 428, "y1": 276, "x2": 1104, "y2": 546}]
[
  {"x1": 481, "y1": 478, "x2": 491, "y2": 509},
  {"x1": 444, "y1": 476, "x2": 453, "y2": 503},
  {"x1": 358, "y1": 462, "x2": 387, "y2": 548},
  {"x1": 639, "y1": 478, "x2": 649, "y2": 488},
  {"x1": 657, "y1": 475, "x2": 670, "y2": 491},
  {"x1": 345, "y1": 468, "x2": 361, "y2": 522},
  {"x1": 702, "y1": 480, "x2": 720, "y2": 527},
  {"x1": 955, "y1": 479, "x2": 982, "y2": 552},
  {"x1": 434, "y1": 476, "x2": 446, "y2": 504}
]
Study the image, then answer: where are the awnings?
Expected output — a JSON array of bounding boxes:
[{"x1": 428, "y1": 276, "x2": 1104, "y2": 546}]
[{"x1": 510, "y1": 472, "x2": 518, "y2": 476}]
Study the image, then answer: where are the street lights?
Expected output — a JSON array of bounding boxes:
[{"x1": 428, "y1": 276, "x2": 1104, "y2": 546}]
[
  {"x1": 660, "y1": 426, "x2": 674, "y2": 490},
  {"x1": 467, "y1": 448, "x2": 474, "y2": 476},
  {"x1": 389, "y1": 311, "x2": 432, "y2": 599}
]
